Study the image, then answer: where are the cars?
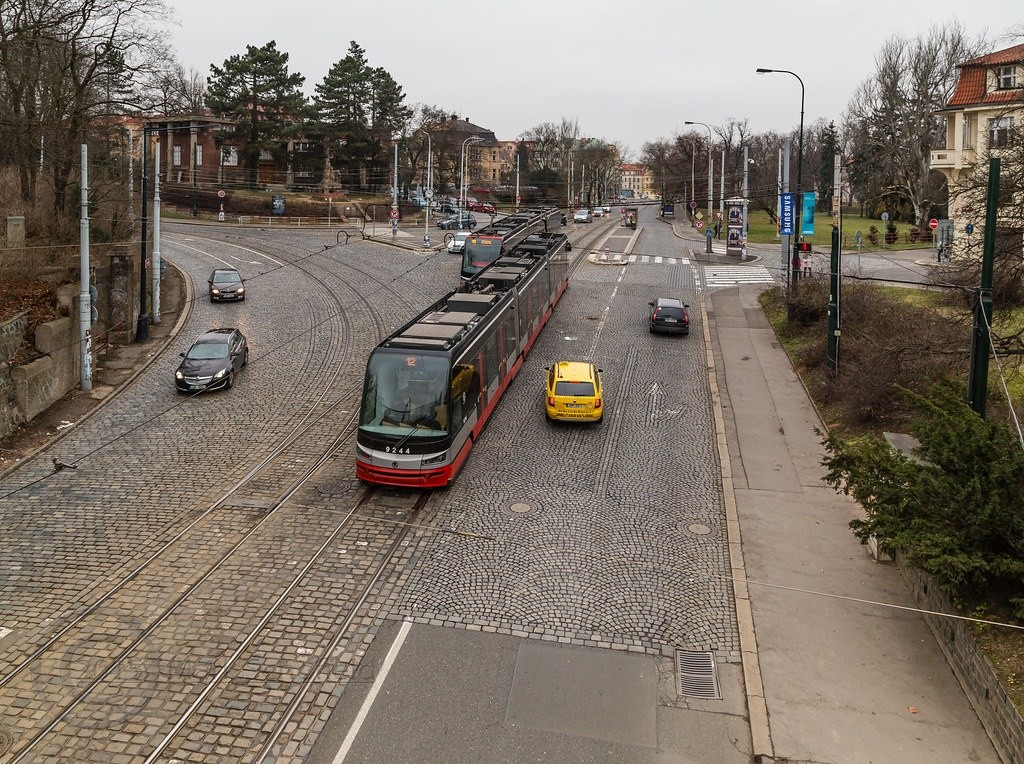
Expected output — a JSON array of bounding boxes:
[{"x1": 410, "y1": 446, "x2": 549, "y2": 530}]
[
  {"x1": 206, "y1": 268, "x2": 248, "y2": 302},
  {"x1": 648, "y1": 296, "x2": 691, "y2": 335},
  {"x1": 173, "y1": 328, "x2": 250, "y2": 393},
  {"x1": 403, "y1": 187, "x2": 495, "y2": 215},
  {"x1": 447, "y1": 230, "x2": 475, "y2": 255},
  {"x1": 543, "y1": 360, "x2": 605, "y2": 425},
  {"x1": 573, "y1": 203, "x2": 611, "y2": 223}
]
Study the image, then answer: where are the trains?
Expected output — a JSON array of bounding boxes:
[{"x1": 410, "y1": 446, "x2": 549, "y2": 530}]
[
  {"x1": 355, "y1": 231, "x2": 572, "y2": 492},
  {"x1": 459, "y1": 203, "x2": 561, "y2": 285}
]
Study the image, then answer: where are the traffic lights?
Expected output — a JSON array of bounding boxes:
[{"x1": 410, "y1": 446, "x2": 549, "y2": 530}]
[{"x1": 796, "y1": 242, "x2": 813, "y2": 253}]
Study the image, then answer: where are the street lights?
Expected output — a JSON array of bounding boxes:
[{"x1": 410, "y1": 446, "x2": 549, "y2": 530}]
[
  {"x1": 676, "y1": 137, "x2": 696, "y2": 227},
  {"x1": 684, "y1": 120, "x2": 713, "y2": 253},
  {"x1": 458, "y1": 137, "x2": 486, "y2": 229},
  {"x1": 420, "y1": 130, "x2": 432, "y2": 248},
  {"x1": 515, "y1": 144, "x2": 530, "y2": 214},
  {"x1": 756, "y1": 68, "x2": 800, "y2": 293},
  {"x1": 135, "y1": 122, "x2": 223, "y2": 343}
]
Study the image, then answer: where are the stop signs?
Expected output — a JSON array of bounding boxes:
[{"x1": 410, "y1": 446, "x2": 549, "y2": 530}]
[
  {"x1": 516, "y1": 196, "x2": 521, "y2": 200},
  {"x1": 929, "y1": 219, "x2": 939, "y2": 229}
]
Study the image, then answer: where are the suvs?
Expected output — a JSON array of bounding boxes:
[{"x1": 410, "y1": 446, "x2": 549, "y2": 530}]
[{"x1": 437, "y1": 214, "x2": 477, "y2": 230}]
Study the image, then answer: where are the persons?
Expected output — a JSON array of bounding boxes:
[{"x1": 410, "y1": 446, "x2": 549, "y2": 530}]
[
  {"x1": 792, "y1": 253, "x2": 813, "y2": 279},
  {"x1": 714, "y1": 221, "x2": 723, "y2": 239},
  {"x1": 936, "y1": 240, "x2": 952, "y2": 263}
]
